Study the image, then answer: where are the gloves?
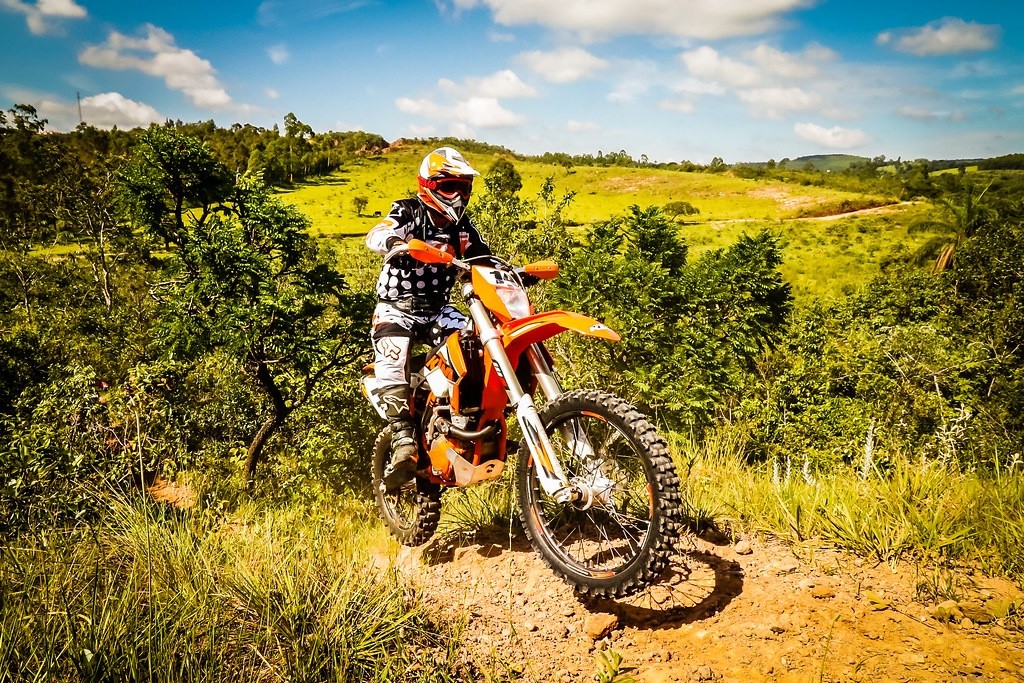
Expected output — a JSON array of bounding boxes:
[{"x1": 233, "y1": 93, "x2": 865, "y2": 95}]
[
  {"x1": 512, "y1": 271, "x2": 541, "y2": 287},
  {"x1": 387, "y1": 236, "x2": 417, "y2": 271}
]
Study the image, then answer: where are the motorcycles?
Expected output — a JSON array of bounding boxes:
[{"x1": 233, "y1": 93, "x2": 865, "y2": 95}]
[{"x1": 359, "y1": 237, "x2": 686, "y2": 600}]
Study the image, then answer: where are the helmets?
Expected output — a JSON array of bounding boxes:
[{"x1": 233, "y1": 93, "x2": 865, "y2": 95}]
[{"x1": 418, "y1": 147, "x2": 480, "y2": 224}]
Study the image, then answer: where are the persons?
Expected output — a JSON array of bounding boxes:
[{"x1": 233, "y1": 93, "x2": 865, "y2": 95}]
[{"x1": 365, "y1": 148, "x2": 539, "y2": 469}]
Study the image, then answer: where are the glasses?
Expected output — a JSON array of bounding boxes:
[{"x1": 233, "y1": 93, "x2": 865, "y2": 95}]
[{"x1": 417, "y1": 176, "x2": 474, "y2": 200}]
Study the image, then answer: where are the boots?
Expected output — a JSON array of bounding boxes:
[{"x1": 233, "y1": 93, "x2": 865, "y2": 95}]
[{"x1": 373, "y1": 386, "x2": 419, "y2": 476}]
